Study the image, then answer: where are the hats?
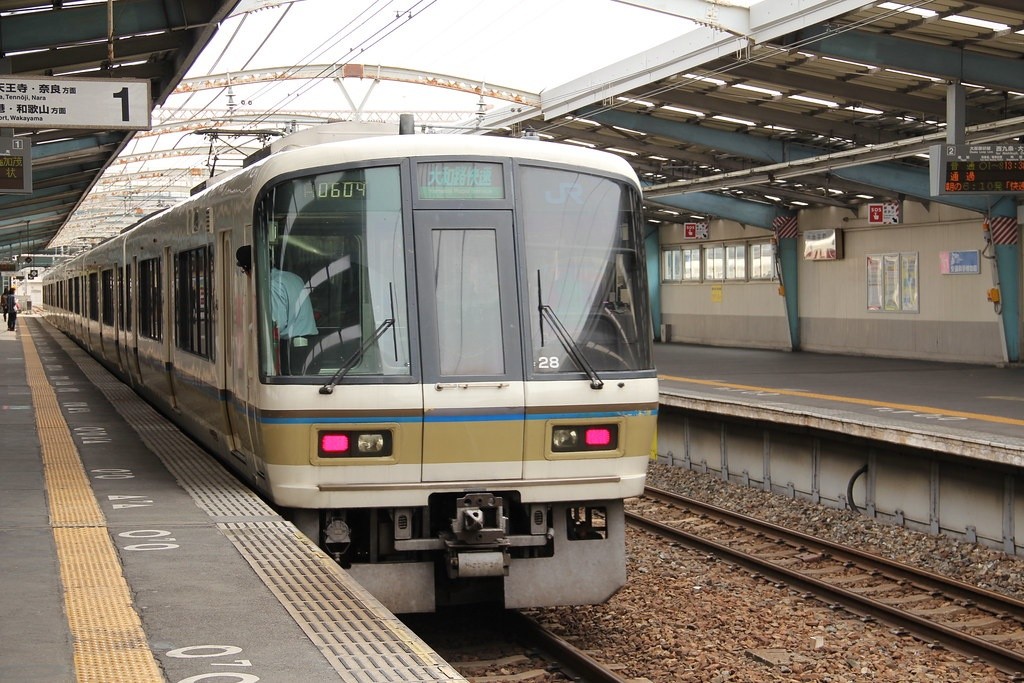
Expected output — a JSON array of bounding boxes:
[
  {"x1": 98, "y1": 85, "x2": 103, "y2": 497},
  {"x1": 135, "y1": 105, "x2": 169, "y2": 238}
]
[{"x1": 236, "y1": 245, "x2": 251, "y2": 266}]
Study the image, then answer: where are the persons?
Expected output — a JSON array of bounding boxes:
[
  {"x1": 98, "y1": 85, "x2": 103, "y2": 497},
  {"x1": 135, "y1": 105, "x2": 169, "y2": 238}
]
[
  {"x1": 7, "y1": 288, "x2": 18, "y2": 331},
  {"x1": 1, "y1": 288, "x2": 8, "y2": 322},
  {"x1": 233, "y1": 243, "x2": 322, "y2": 377}
]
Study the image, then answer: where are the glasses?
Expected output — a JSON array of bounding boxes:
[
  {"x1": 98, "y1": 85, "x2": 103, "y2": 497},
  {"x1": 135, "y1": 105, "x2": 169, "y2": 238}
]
[{"x1": 239, "y1": 266, "x2": 250, "y2": 273}]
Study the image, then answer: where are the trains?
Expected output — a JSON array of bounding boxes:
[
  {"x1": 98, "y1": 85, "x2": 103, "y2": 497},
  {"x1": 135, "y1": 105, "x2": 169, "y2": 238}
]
[{"x1": 41, "y1": 112, "x2": 658, "y2": 613}]
